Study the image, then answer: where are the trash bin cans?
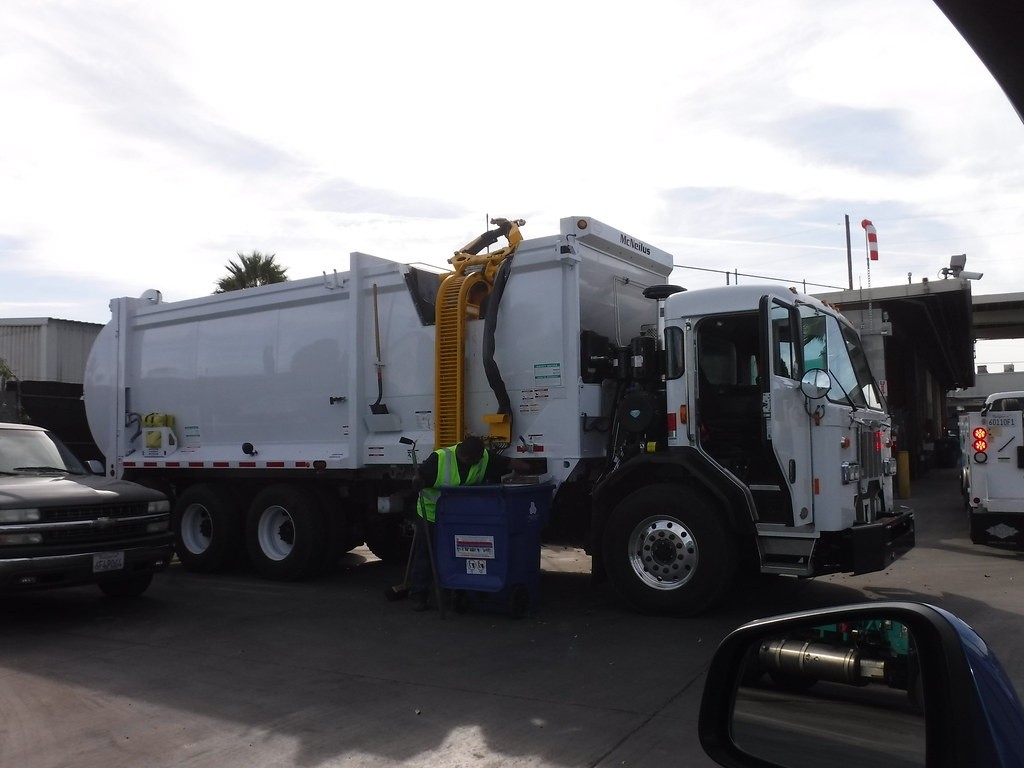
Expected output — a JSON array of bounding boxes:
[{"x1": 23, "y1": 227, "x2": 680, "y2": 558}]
[{"x1": 434, "y1": 481, "x2": 555, "y2": 620}]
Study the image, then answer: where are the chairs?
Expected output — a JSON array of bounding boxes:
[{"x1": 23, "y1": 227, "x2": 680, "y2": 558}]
[{"x1": 698, "y1": 337, "x2": 762, "y2": 417}]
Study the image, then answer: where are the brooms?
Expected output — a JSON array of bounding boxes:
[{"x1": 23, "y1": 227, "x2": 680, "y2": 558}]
[{"x1": 384, "y1": 524, "x2": 417, "y2": 600}]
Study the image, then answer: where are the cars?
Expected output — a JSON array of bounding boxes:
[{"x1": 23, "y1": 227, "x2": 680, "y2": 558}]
[{"x1": 0, "y1": 423, "x2": 178, "y2": 597}]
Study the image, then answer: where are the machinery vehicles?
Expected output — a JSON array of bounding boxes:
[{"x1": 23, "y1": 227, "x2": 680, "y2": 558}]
[
  {"x1": 79, "y1": 214, "x2": 916, "y2": 613},
  {"x1": 742, "y1": 619, "x2": 924, "y2": 713},
  {"x1": 956, "y1": 390, "x2": 1023, "y2": 544}
]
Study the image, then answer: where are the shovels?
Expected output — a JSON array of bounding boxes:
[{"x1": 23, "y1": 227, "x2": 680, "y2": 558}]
[{"x1": 368, "y1": 285, "x2": 388, "y2": 416}]
